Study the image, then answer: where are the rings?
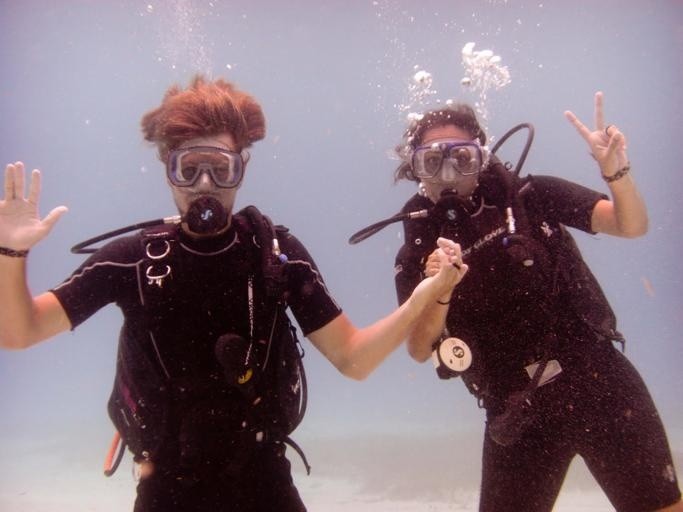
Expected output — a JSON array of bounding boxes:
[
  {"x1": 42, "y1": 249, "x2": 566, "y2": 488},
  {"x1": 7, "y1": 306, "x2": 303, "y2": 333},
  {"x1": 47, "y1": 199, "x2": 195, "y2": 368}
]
[{"x1": 453, "y1": 262, "x2": 461, "y2": 270}]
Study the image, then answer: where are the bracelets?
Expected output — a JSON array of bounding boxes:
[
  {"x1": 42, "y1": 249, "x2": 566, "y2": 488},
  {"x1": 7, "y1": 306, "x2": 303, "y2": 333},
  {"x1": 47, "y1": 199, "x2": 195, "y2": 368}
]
[
  {"x1": 437, "y1": 301, "x2": 451, "y2": 305},
  {"x1": 600, "y1": 160, "x2": 631, "y2": 182},
  {"x1": 0, "y1": 247, "x2": 29, "y2": 258}
]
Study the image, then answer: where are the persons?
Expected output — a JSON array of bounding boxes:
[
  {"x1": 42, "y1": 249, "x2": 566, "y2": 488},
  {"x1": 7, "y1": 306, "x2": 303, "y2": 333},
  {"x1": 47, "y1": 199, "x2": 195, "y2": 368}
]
[
  {"x1": 396, "y1": 91, "x2": 682, "y2": 511},
  {"x1": 0, "y1": 69, "x2": 469, "y2": 511}
]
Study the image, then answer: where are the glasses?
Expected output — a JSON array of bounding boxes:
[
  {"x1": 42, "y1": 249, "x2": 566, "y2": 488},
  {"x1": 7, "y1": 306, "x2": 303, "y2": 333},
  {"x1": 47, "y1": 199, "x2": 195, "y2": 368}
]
[
  {"x1": 410, "y1": 141, "x2": 481, "y2": 179},
  {"x1": 166, "y1": 144, "x2": 245, "y2": 190}
]
